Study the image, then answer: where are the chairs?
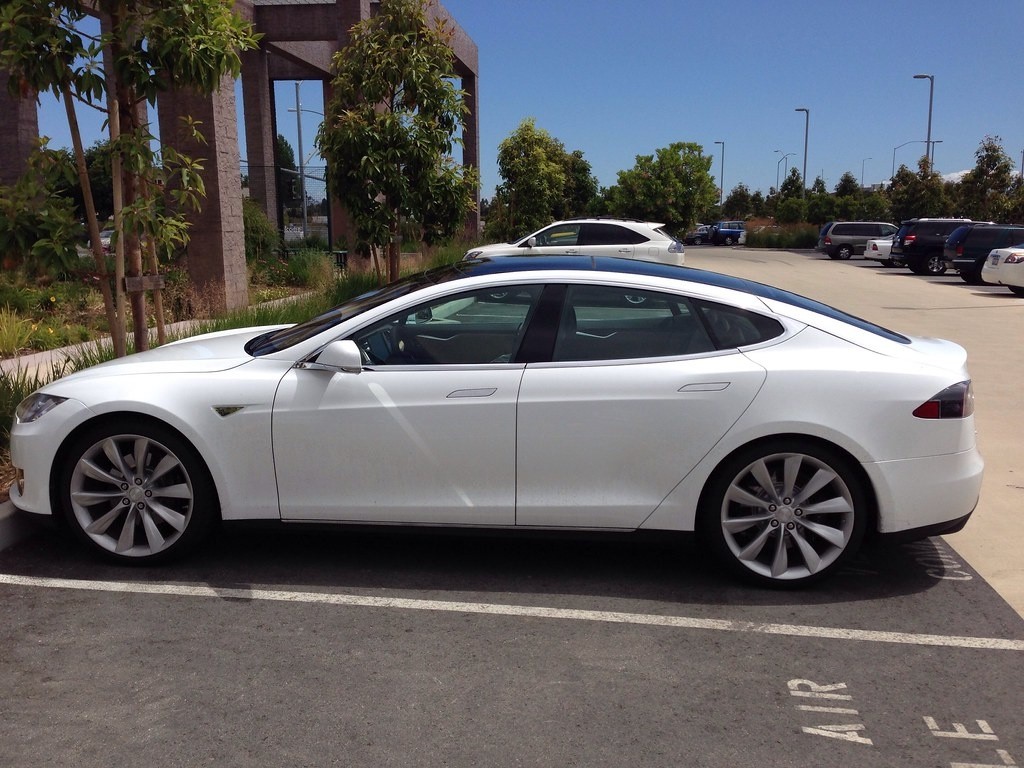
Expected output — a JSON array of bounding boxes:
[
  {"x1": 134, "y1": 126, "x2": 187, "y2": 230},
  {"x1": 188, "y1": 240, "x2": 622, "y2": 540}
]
[{"x1": 557, "y1": 305, "x2": 577, "y2": 362}]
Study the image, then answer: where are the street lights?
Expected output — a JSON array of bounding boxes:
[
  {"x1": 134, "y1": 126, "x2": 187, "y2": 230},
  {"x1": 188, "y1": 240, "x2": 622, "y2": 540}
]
[
  {"x1": 775, "y1": 152, "x2": 797, "y2": 192},
  {"x1": 862, "y1": 157, "x2": 872, "y2": 185},
  {"x1": 892, "y1": 140, "x2": 932, "y2": 177},
  {"x1": 925, "y1": 139, "x2": 944, "y2": 173},
  {"x1": 794, "y1": 107, "x2": 810, "y2": 202},
  {"x1": 912, "y1": 73, "x2": 936, "y2": 160},
  {"x1": 714, "y1": 141, "x2": 724, "y2": 208},
  {"x1": 773, "y1": 149, "x2": 788, "y2": 180}
]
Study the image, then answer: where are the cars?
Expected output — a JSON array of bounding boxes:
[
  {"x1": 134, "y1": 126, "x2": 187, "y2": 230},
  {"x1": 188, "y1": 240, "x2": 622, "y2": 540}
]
[
  {"x1": 980, "y1": 242, "x2": 1024, "y2": 299},
  {"x1": 752, "y1": 225, "x2": 792, "y2": 237},
  {"x1": 9, "y1": 251, "x2": 990, "y2": 590},
  {"x1": 87, "y1": 229, "x2": 116, "y2": 251},
  {"x1": 864, "y1": 232, "x2": 906, "y2": 268}
]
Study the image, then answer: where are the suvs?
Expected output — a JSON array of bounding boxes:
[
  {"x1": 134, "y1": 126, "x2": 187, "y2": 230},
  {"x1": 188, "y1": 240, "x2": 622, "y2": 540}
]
[
  {"x1": 888, "y1": 218, "x2": 999, "y2": 274},
  {"x1": 814, "y1": 221, "x2": 900, "y2": 260},
  {"x1": 461, "y1": 215, "x2": 686, "y2": 311},
  {"x1": 941, "y1": 222, "x2": 1024, "y2": 284},
  {"x1": 683, "y1": 220, "x2": 746, "y2": 245}
]
